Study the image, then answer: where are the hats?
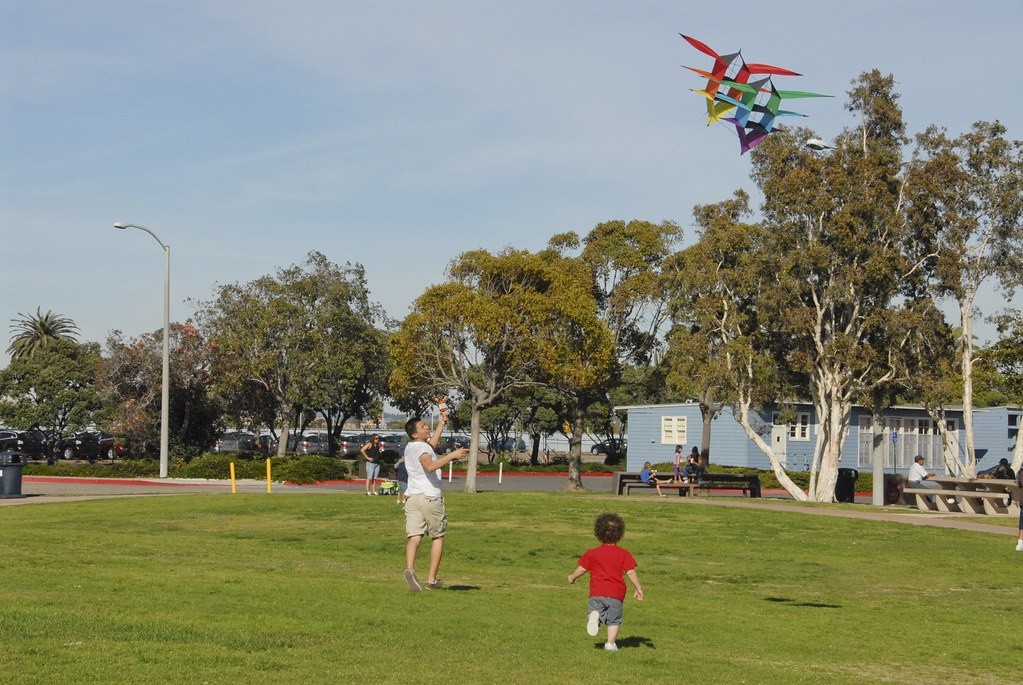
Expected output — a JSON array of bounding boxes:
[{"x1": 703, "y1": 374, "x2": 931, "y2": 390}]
[
  {"x1": 998, "y1": 458, "x2": 1008, "y2": 464},
  {"x1": 915, "y1": 455, "x2": 926, "y2": 459}
]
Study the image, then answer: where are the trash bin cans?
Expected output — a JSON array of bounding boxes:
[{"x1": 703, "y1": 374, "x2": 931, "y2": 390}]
[
  {"x1": 0, "y1": 452, "x2": 27, "y2": 500},
  {"x1": 835, "y1": 468, "x2": 859, "y2": 503}
]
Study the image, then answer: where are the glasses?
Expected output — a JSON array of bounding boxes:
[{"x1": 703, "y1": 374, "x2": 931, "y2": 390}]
[{"x1": 375, "y1": 438, "x2": 379, "y2": 440}]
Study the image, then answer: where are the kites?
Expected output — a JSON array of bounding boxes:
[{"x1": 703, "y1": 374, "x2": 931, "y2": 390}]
[{"x1": 679, "y1": 33, "x2": 836, "y2": 155}]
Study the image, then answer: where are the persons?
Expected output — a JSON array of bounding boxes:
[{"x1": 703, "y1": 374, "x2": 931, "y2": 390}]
[
  {"x1": 908, "y1": 455, "x2": 936, "y2": 489},
  {"x1": 683, "y1": 446, "x2": 702, "y2": 484},
  {"x1": 361, "y1": 434, "x2": 382, "y2": 496},
  {"x1": 994, "y1": 458, "x2": 1016, "y2": 480},
  {"x1": 1015, "y1": 462, "x2": 1023, "y2": 551},
  {"x1": 394, "y1": 455, "x2": 409, "y2": 504},
  {"x1": 403, "y1": 413, "x2": 471, "y2": 591},
  {"x1": 673, "y1": 445, "x2": 683, "y2": 484},
  {"x1": 641, "y1": 462, "x2": 673, "y2": 496},
  {"x1": 568, "y1": 514, "x2": 645, "y2": 650}
]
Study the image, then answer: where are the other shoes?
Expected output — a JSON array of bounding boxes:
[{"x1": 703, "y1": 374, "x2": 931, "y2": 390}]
[
  {"x1": 667, "y1": 478, "x2": 673, "y2": 484},
  {"x1": 372, "y1": 492, "x2": 379, "y2": 495},
  {"x1": 396, "y1": 499, "x2": 400, "y2": 504},
  {"x1": 404, "y1": 569, "x2": 422, "y2": 593},
  {"x1": 424, "y1": 579, "x2": 443, "y2": 591},
  {"x1": 586, "y1": 610, "x2": 600, "y2": 637},
  {"x1": 367, "y1": 492, "x2": 372, "y2": 495},
  {"x1": 660, "y1": 494, "x2": 667, "y2": 498},
  {"x1": 604, "y1": 643, "x2": 617, "y2": 650},
  {"x1": 683, "y1": 480, "x2": 690, "y2": 484},
  {"x1": 402, "y1": 501, "x2": 405, "y2": 504},
  {"x1": 1015, "y1": 545, "x2": 1023, "y2": 552}
]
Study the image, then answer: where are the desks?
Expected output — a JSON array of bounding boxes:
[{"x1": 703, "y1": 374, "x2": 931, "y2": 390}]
[
  {"x1": 922, "y1": 476, "x2": 1023, "y2": 515},
  {"x1": 610, "y1": 470, "x2": 762, "y2": 498}
]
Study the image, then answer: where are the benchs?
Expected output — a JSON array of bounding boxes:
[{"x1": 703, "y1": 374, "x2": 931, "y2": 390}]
[
  {"x1": 625, "y1": 483, "x2": 757, "y2": 498},
  {"x1": 903, "y1": 488, "x2": 1010, "y2": 516}
]
